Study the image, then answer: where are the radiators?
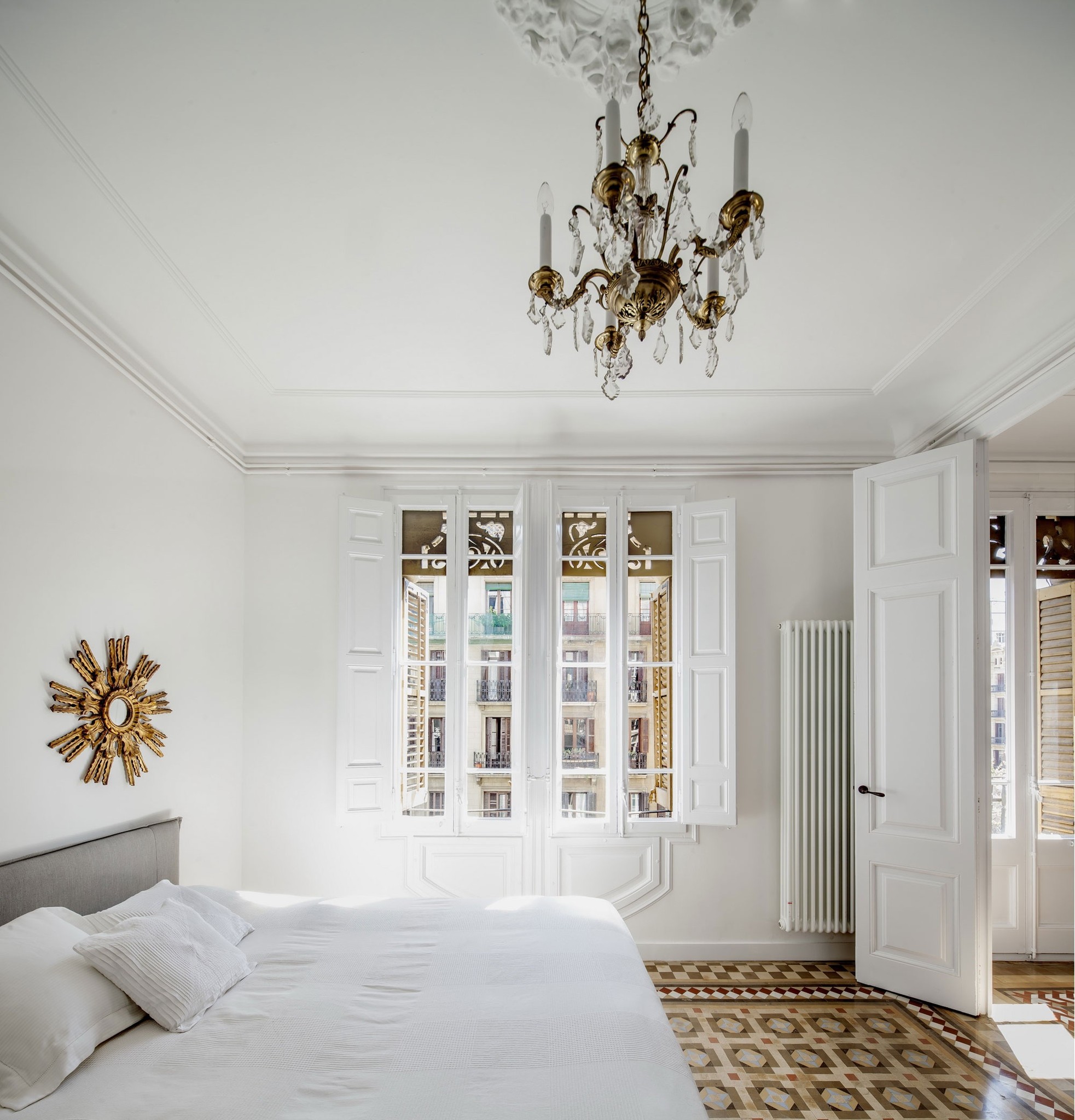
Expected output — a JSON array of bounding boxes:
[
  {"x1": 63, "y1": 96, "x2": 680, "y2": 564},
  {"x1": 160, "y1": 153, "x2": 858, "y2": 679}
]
[{"x1": 775, "y1": 619, "x2": 864, "y2": 932}]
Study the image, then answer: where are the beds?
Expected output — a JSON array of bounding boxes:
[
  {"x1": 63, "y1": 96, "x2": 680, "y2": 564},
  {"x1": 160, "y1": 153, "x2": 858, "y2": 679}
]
[{"x1": 0, "y1": 809, "x2": 714, "y2": 1120}]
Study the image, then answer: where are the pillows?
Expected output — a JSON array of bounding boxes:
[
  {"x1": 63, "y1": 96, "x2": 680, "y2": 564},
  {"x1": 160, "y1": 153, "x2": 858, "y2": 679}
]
[
  {"x1": 0, "y1": 906, "x2": 142, "y2": 1113},
  {"x1": 71, "y1": 873, "x2": 270, "y2": 1026}
]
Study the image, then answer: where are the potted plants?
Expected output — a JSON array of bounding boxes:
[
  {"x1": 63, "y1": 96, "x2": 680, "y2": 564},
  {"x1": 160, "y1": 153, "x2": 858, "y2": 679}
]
[
  {"x1": 480, "y1": 606, "x2": 511, "y2": 634},
  {"x1": 563, "y1": 746, "x2": 590, "y2": 759}
]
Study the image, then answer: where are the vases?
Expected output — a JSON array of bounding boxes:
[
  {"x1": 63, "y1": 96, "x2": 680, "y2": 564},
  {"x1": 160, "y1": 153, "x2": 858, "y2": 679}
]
[{"x1": 476, "y1": 763, "x2": 482, "y2": 768}]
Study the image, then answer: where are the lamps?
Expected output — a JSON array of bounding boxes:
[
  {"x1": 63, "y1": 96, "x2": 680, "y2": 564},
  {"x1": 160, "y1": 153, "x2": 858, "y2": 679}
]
[{"x1": 512, "y1": 0, "x2": 799, "y2": 407}]
